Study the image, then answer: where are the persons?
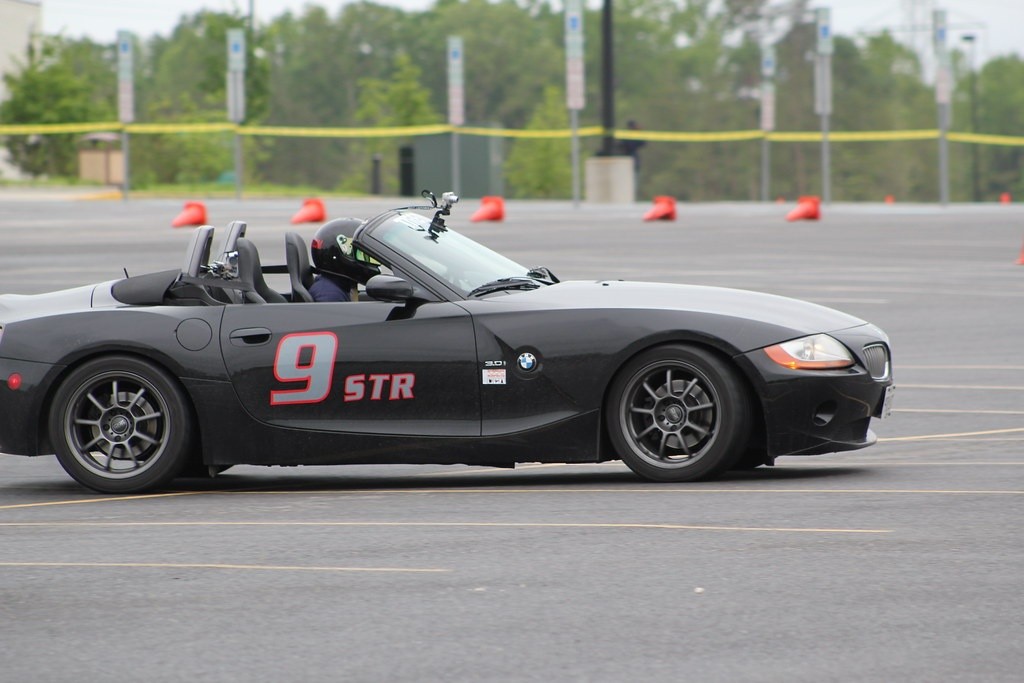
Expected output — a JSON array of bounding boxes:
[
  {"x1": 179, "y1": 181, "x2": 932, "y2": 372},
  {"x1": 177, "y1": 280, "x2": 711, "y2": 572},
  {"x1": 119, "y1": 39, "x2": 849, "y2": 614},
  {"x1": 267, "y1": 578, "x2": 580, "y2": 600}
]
[{"x1": 308, "y1": 217, "x2": 381, "y2": 300}]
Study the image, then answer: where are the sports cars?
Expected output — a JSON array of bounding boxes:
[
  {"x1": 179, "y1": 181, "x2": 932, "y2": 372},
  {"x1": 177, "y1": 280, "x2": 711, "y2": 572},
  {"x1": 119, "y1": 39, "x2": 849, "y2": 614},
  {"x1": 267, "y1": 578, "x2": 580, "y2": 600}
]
[{"x1": 0, "y1": 188, "x2": 895, "y2": 496}]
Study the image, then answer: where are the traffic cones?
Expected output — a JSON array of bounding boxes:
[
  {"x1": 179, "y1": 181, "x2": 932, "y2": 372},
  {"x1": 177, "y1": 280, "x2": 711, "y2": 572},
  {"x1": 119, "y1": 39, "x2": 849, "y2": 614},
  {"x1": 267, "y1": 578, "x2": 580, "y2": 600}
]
[
  {"x1": 1014, "y1": 240, "x2": 1024, "y2": 265},
  {"x1": 172, "y1": 201, "x2": 207, "y2": 229},
  {"x1": 1000, "y1": 192, "x2": 1011, "y2": 203},
  {"x1": 291, "y1": 196, "x2": 326, "y2": 226},
  {"x1": 785, "y1": 194, "x2": 821, "y2": 222},
  {"x1": 644, "y1": 194, "x2": 676, "y2": 223},
  {"x1": 469, "y1": 196, "x2": 504, "y2": 225}
]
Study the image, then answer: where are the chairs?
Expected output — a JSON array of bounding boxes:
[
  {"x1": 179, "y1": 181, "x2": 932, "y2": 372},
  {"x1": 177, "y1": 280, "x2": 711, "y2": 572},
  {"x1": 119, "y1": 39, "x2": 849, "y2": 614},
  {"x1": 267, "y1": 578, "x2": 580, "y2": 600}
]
[
  {"x1": 235, "y1": 239, "x2": 289, "y2": 304},
  {"x1": 284, "y1": 233, "x2": 315, "y2": 303}
]
